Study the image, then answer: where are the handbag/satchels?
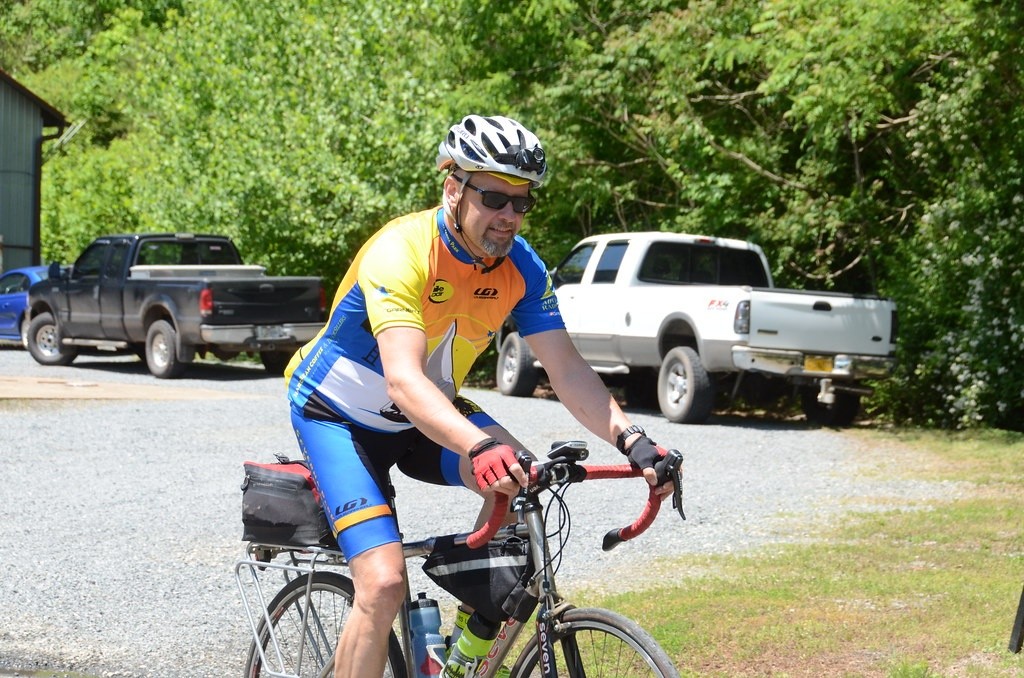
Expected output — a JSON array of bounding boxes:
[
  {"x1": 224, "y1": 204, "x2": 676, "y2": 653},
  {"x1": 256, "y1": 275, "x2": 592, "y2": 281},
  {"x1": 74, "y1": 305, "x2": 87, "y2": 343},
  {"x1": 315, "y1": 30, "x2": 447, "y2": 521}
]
[{"x1": 241, "y1": 452, "x2": 399, "y2": 550}]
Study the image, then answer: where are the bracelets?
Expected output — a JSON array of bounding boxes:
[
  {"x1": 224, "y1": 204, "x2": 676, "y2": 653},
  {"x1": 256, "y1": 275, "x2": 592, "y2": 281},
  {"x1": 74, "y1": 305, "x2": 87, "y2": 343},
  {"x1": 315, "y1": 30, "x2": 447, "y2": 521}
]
[{"x1": 616, "y1": 424, "x2": 646, "y2": 455}]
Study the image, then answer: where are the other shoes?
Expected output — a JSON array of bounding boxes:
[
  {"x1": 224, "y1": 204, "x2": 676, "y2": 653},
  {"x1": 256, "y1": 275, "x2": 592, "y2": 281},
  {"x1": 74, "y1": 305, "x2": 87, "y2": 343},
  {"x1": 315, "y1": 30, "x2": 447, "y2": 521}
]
[{"x1": 445, "y1": 635, "x2": 510, "y2": 678}]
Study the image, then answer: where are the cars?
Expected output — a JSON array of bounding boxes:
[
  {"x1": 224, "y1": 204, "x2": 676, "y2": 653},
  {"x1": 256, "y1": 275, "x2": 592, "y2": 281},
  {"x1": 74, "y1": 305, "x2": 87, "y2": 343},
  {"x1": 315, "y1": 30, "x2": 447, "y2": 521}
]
[{"x1": 0, "y1": 263, "x2": 69, "y2": 341}]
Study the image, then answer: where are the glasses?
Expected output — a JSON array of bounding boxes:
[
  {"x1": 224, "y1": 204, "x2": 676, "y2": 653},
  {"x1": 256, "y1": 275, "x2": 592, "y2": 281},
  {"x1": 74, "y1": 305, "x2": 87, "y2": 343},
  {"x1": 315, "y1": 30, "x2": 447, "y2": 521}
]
[{"x1": 452, "y1": 172, "x2": 536, "y2": 213}]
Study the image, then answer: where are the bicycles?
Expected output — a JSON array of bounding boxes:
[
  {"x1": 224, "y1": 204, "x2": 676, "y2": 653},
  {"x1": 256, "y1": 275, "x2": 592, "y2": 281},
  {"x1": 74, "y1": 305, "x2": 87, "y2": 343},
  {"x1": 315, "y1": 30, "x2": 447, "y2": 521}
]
[{"x1": 234, "y1": 448, "x2": 686, "y2": 678}]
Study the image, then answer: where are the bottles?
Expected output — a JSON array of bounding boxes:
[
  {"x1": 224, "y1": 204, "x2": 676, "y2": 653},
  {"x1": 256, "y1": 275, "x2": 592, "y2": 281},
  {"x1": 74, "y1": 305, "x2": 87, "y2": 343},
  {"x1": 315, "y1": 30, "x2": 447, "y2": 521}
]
[
  {"x1": 439, "y1": 610, "x2": 502, "y2": 678},
  {"x1": 408, "y1": 592, "x2": 447, "y2": 678}
]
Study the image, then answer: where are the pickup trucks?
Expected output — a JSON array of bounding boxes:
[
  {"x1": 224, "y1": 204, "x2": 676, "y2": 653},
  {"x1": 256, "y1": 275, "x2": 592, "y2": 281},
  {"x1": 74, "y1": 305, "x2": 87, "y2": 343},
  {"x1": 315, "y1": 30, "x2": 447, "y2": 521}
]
[
  {"x1": 27, "y1": 231, "x2": 329, "y2": 379},
  {"x1": 496, "y1": 231, "x2": 901, "y2": 426}
]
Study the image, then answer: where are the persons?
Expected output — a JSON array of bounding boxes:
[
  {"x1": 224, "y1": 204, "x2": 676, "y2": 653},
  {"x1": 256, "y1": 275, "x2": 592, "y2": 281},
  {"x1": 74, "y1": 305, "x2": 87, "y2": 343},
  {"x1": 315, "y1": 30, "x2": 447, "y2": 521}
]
[{"x1": 286, "y1": 115, "x2": 682, "y2": 678}]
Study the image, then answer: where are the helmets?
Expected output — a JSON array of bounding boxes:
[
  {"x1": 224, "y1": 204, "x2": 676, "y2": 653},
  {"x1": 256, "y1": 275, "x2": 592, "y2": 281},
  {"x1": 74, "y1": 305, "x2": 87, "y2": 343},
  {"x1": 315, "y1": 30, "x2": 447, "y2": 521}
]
[{"x1": 436, "y1": 114, "x2": 546, "y2": 189}]
